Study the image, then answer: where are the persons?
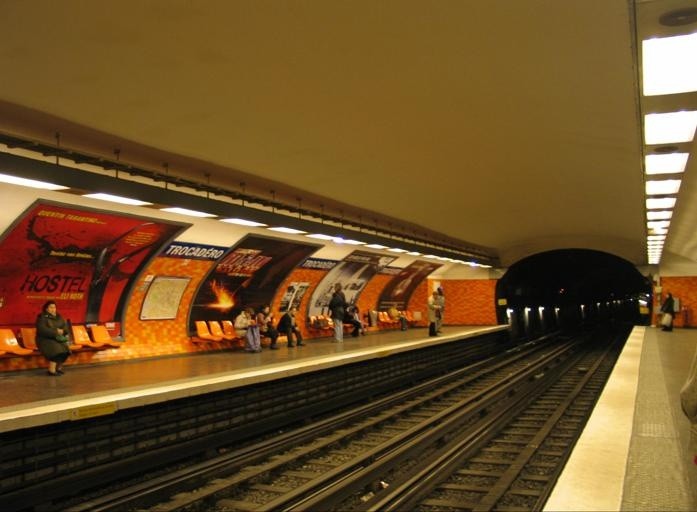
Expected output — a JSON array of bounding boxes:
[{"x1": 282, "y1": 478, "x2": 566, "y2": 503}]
[
  {"x1": 279, "y1": 286, "x2": 294, "y2": 312},
  {"x1": 435, "y1": 286, "x2": 446, "y2": 333},
  {"x1": 327, "y1": 283, "x2": 344, "y2": 343},
  {"x1": 232, "y1": 307, "x2": 263, "y2": 353},
  {"x1": 388, "y1": 302, "x2": 407, "y2": 331},
  {"x1": 253, "y1": 303, "x2": 280, "y2": 349},
  {"x1": 342, "y1": 302, "x2": 362, "y2": 336},
  {"x1": 275, "y1": 305, "x2": 305, "y2": 347},
  {"x1": 660, "y1": 292, "x2": 675, "y2": 331},
  {"x1": 426, "y1": 291, "x2": 441, "y2": 336},
  {"x1": 352, "y1": 306, "x2": 367, "y2": 336},
  {"x1": 34, "y1": 299, "x2": 71, "y2": 377}
]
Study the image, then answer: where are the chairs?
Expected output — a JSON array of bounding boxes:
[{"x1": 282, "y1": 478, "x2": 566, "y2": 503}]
[
  {"x1": 307, "y1": 314, "x2": 367, "y2": 331},
  {"x1": 377, "y1": 310, "x2": 422, "y2": 325},
  {"x1": 194, "y1": 320, "x2": 286, "y2": 342},
  {"x1": 0, "y1": 325, "x2": 123, "y2": 359}
]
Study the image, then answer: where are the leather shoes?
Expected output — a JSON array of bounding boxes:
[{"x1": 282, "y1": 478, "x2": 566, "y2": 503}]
[{"x1": 46, "y1": 368, "x2": 64, "y2": 377}]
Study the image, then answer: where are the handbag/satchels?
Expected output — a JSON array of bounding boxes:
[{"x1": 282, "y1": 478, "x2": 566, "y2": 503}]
[{"x1": 55, "y1": 332, "x2": 69, "y2": 343}]
